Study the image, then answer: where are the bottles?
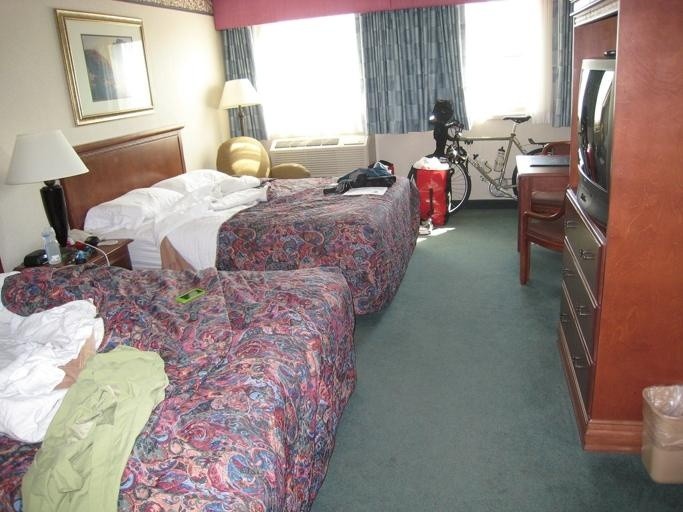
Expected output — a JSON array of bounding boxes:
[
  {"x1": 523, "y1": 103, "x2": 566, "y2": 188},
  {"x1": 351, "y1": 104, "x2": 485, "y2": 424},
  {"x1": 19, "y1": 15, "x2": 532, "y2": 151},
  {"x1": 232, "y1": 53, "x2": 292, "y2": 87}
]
[
  {"x1": 472, "y1": 153, "x2": 492, "y2": 173},
  {"x1": 493, "y1": 146, "x2": 505, "y2": 173},
  {"x1": 40, "y1": 222, "x2": 62, "y2": 266}
]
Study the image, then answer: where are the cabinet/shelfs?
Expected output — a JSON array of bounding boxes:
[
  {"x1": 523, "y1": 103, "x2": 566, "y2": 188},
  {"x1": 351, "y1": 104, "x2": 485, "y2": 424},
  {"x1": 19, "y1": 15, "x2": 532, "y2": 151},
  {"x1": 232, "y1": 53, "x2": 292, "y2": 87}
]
[{"x1": 558, "y1": 0, "x2": 683, "y2": 454}]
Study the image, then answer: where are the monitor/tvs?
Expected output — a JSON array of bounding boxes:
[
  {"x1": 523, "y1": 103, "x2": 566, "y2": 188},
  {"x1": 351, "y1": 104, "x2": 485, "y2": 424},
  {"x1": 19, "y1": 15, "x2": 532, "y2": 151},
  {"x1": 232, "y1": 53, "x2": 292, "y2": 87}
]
[{"x1": 577, "y1": 58, "x2": 615, "y2": 230}]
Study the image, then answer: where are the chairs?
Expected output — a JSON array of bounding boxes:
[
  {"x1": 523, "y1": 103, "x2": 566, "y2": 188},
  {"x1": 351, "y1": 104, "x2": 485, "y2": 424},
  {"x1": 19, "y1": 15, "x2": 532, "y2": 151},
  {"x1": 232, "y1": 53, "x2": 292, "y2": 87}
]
[{"x1": 269, "y1": 163, "x2": 310, "y2": 179}]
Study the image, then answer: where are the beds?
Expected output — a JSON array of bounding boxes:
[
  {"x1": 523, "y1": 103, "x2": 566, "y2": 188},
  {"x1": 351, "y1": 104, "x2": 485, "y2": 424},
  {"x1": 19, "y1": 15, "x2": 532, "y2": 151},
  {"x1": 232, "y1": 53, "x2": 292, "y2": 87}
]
[
  {"x1": 58, "y1": 126, "x2": 419, "y2": 315},
  {"x1": 1, "y1": 267, "x2": 358, "y2": 512}
]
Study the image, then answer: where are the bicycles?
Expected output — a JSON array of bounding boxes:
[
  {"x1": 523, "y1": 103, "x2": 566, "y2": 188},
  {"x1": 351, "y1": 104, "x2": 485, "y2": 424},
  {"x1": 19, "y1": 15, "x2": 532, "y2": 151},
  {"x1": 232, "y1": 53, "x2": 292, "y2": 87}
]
[{"x1": 408, "y1": 115, "x2": 552, "y2": 214}]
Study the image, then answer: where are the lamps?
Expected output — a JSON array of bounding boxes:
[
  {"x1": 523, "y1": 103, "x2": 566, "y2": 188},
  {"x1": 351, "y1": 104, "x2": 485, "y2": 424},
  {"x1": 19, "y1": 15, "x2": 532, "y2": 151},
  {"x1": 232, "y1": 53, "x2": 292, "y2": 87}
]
[
  {"x1": 216, "y1": 135, "x2": 270, "y2": 178},
  {"x1": 5, "y1": 127, "x2": 90, "y2": 248},
  {"x1": 218, "y1": 78, "x2": 261, "y2": 135}
]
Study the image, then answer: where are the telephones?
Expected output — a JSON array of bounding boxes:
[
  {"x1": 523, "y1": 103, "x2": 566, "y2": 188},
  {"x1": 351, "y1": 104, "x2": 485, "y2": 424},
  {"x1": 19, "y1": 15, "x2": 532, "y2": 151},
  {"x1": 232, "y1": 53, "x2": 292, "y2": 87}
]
[{"x1": 68, "y1": 228, "x2": 95, "y2": 245}]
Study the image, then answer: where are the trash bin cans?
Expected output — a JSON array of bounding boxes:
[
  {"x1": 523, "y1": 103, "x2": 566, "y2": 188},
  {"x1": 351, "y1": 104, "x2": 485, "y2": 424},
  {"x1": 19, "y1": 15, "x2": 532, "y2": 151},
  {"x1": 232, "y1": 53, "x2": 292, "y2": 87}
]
[{"x1": 640, "y1": 385, "x2": 682, "y2": 485}]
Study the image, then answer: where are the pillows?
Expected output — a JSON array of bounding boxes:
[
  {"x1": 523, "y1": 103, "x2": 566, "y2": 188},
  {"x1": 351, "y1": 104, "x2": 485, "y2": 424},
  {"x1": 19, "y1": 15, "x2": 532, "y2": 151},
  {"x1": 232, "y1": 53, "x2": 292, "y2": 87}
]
[
  {"x1": 154, "y1": 168, "x2": 234, "y2": 194},
  {"x1": 80, "y1": 187, "x2": 182, "y2": 231}
]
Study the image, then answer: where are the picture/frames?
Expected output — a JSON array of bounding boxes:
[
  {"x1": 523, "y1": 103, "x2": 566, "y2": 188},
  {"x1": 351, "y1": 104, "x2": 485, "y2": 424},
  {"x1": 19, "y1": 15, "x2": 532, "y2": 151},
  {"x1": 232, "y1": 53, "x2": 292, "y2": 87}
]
[{"x1": 53, "y1": 7, "x2": 157, "y2": 126}]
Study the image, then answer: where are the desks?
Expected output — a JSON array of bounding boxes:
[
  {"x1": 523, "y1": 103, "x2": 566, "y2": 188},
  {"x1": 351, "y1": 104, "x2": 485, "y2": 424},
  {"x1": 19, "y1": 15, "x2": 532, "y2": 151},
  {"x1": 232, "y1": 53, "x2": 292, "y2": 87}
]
[{"x1": 514, "y1": 154, "x2": 570, "y2": 286}]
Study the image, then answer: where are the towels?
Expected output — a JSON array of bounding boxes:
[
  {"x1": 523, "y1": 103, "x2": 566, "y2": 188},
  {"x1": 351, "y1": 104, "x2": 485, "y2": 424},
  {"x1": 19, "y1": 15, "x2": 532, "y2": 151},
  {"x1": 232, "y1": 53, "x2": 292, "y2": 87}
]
[{"x1": 210, "y1": 176, "x2": 268, "y2": 212}]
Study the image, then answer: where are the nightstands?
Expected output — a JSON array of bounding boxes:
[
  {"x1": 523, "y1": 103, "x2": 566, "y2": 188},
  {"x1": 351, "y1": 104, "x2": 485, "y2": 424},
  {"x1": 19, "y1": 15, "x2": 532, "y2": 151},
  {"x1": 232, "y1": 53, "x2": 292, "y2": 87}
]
[{"x1": 28, "y1": 237, "x2": 133, "y2": 266}]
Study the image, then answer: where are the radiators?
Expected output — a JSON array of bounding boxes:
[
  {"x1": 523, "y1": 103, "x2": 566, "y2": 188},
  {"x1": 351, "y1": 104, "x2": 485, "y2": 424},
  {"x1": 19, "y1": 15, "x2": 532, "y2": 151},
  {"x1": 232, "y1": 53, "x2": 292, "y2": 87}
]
[{"x1": 270, "y1": 134, "x2": 368, "y2": 178}]
